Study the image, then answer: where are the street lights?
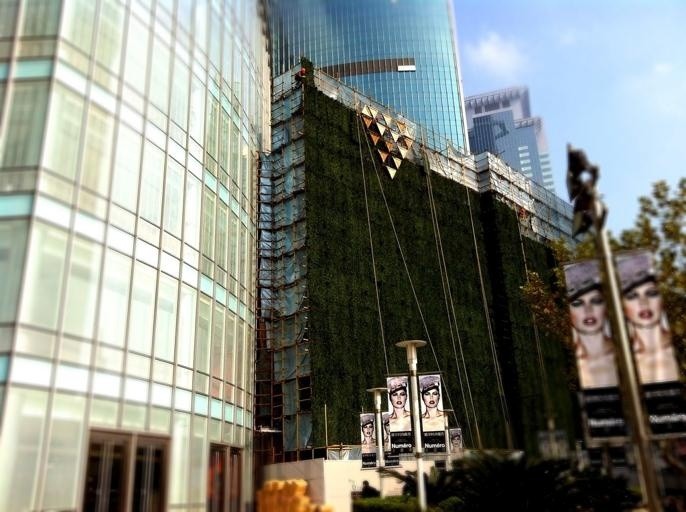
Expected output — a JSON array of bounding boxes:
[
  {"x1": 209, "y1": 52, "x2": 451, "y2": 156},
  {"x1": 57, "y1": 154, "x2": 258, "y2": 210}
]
[
  {"x1": 566, "y1": 143, "x2": 665, "y2": 512},
  {"x1": 396, "y1": 340, "x2": 427, "y2": 512},
  {"x1": 443, "y1": 409, "x2": 455, "y2": 464},
  {"x1": 366, "y1": 387, "x2": 388, "y2": 499}
]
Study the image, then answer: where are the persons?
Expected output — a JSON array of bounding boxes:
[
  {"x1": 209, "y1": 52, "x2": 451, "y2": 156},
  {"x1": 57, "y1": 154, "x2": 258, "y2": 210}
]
[
  {"x1": 418, "y1": 376, "x2": 448, "y2": 431},
  {"x1": 388, "y1": 378, "x2": 411, "y2": 431},
  {"x1": 384, "y1": 416, "x2": 391, "y2": 449},
  {"x1": 615, "y1": 255, "x2": 679, "y2": 382},
  {"x1": 363, "y1": 418, "x2": 376, "y2": 448},
  {"x1": 564, "y1": 263, "x2": 618, "y2": 391},
  {"x1": 450, "y1": 432, "x2": 460, "y2": 454}
]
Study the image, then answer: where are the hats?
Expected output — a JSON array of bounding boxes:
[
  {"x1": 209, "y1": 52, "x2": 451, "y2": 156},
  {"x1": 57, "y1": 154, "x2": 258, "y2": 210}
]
[
  {"x1": 419, "y1": 376, "x2": 439, "y2": 393},
  {"x1": 615, "y1": 253, "x2": 654, "y2": 292},
  {"x1": 382, "y1": 414, "x2": 388, "y2": 424},
  {"x1": 564, "y1": 262, "x2": 603, "y2": 302},
  {"x1": 451, "y1": 433, "x2": 459, "y2": 439},
  {"x1": 387, "y1": 378, "x2": 407, "y2": 393},
  {"x1": 361, "y1": 415, "x2": 373, "y2": 425}
]
